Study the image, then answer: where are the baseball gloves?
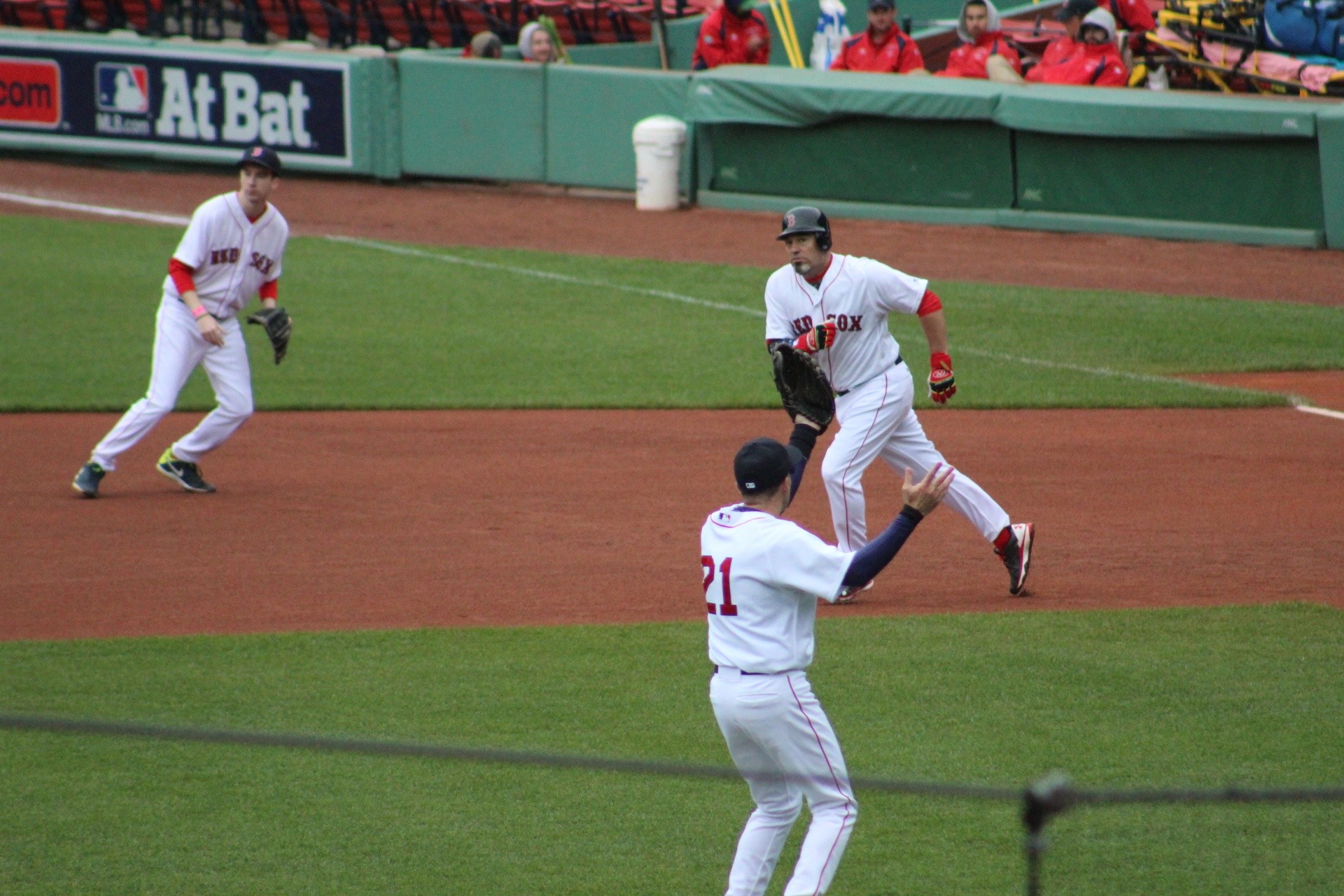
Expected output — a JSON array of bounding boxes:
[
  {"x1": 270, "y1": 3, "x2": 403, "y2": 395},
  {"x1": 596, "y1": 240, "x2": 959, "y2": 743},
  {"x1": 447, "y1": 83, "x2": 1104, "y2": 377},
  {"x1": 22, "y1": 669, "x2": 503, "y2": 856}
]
[
  {"x1": 771, "y1": 343, "x2": 837, "y2": 434},
  {"x1": 246, "y1": 306, "x2": 290, "y2": 367}
]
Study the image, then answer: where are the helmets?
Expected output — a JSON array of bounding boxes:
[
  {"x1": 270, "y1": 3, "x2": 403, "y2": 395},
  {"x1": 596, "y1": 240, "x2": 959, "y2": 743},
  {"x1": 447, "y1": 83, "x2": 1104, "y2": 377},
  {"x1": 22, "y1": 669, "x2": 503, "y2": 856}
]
[{"x1": 776, "y1": 206, "x2": 831, "y2": 253}]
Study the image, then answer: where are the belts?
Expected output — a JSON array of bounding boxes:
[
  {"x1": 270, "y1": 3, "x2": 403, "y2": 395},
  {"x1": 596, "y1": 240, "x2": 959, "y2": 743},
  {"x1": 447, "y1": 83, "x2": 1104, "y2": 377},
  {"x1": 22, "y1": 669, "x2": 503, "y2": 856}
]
[
  {"x1": 838, "y1": 355, "x2": 905, "y2": 395},
  {"x1": 714, "y1": 663, "x2": 760, "y2": 675}
]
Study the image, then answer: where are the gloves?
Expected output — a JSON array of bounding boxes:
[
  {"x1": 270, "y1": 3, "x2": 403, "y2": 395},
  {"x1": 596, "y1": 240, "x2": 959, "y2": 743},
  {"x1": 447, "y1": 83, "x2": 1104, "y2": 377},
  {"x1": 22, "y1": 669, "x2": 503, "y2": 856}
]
[
  {"x1": 791, "y1": 321, "x2": 839, "y2": 356},
  {"x1": 928, "y1": 352, "x2": 957, "y2": 405}
]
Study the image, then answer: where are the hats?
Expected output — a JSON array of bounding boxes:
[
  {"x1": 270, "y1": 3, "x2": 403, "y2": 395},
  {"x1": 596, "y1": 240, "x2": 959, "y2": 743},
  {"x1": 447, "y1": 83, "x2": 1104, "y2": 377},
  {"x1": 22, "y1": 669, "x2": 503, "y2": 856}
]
[
  {"x1": 866, "y1": 0, "x2": 893, "y2": 12},
  {"x1": 235, "y1": 145, "x2": 281, "y2": 178},
  {"x1": 734, "y1": 435, "x2": 803, "y2": 495}
]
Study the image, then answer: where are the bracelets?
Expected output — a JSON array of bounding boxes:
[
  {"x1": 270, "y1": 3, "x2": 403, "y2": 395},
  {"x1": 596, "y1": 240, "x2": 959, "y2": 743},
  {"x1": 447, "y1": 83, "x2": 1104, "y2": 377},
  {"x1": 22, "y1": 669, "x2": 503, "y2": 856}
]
[{"x1": 192, "y1": 306, "x2": 207, "y2": 319}]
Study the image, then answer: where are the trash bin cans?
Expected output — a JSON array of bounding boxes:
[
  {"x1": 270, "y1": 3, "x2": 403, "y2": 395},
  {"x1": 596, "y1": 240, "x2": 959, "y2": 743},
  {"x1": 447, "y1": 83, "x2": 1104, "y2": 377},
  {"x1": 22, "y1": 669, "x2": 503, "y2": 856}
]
[{"x1": 631, "y1": 113, "x2": 688, "y2": 211}]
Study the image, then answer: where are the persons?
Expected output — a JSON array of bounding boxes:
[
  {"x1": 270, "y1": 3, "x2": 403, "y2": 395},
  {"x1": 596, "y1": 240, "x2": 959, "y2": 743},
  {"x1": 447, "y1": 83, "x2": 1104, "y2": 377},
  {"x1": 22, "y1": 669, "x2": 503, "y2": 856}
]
[
  {"x1": 700, "y1": 344, "x2": 957, "y2": 896},
  {"x1": 910, "y1": 0, "x2": 1021, "y2": 82},
  {"x1": 692, "y1": 0, "x2": 769, "y2": 71},
  {"x1": 517, "y1": 22, "x2": 557, "y2": 64},
  {"x1": 764, "y1": 206, "x2": 1036, "y2": 593},
  {"x1": 986, "y1": 9, "x2": 1129, "y2": 89},
  {"x1": 829, "y1": 1, "x2": 925, "y2": 77},
  {"x1": 71, "y1": 147, "x2": 292, "y2": 497},
  {"x1": 471, "y1": 31, "x2": 504, "y2": 58},
  {"x1": 1041, "y1": 0, "x2": 1097, "y2": 64}
]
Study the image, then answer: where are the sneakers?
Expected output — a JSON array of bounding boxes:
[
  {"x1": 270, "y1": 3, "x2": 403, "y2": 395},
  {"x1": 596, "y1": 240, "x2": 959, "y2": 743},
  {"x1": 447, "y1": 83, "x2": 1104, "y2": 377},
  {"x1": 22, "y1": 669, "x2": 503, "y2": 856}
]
[
  {"x1": 835, "y1": 577, "x2": 877, "y2": 603},
  {"x1": 994, "y1": 519, "x2": 1036, "y2": 594},
  {"x1": 72, "y1": 461, "x2": 105, "y2": 499},
  {"x1": 154, "y1": 448, "x2": 216, "y2": 494}
]
